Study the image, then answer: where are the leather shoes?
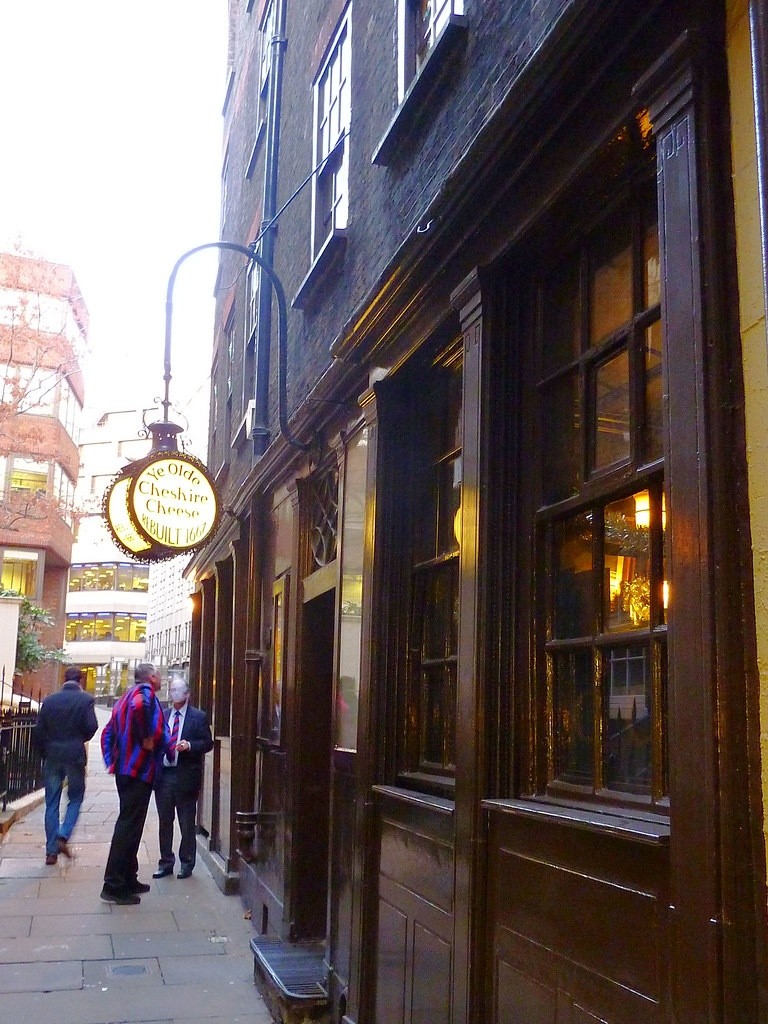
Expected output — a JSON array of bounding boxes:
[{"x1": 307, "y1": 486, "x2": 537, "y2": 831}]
[
  {"x1": 174, "y1": 861, "x2": 193, "y2": 879},
  {"x1": 59, "y1": 846, "x2": 75, "y2": 864},
  {"x1": 47, "y1": 855, "x2": 57, "y2": 866},
  {"x1": 150, "y1": 869, "x2": 175, "y2": 878}
]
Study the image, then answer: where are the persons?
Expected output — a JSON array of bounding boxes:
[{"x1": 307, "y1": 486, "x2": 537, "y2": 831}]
[
  {"x1": 100, "y1": 662, "x2": 215, "y2": 905},
  {"x1": 34, "y1": 667, "x2": 99, "y2": 866}
]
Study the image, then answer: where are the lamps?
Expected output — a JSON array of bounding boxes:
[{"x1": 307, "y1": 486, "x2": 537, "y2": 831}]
[{"x1": 101, "y1": 242, "x2": 324, "y2": 566}]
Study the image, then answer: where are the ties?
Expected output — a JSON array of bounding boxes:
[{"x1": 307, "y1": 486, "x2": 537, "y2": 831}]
[{"x1": 168, "y1": 709, "x2": 182, "y2": 765}]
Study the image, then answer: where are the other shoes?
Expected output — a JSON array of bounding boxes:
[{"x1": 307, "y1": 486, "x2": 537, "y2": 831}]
[
  {"x1": 131, "y1": 879, "x2": 150, "y2": 893},
  {"x1": 99, "y1": 888, "x2": 141, "y2": 906}
]
